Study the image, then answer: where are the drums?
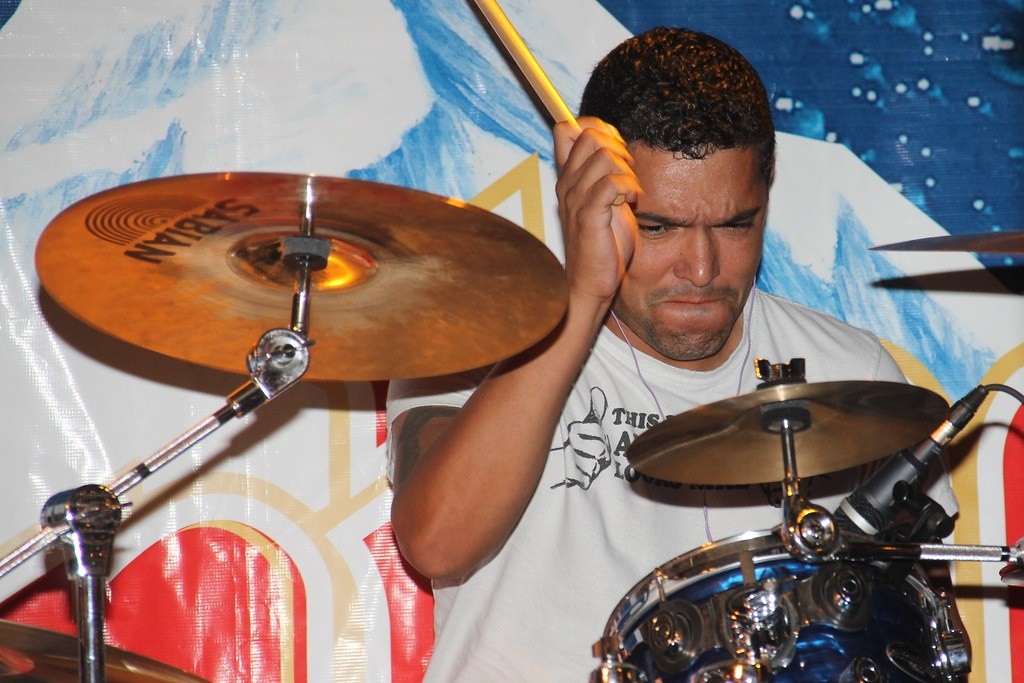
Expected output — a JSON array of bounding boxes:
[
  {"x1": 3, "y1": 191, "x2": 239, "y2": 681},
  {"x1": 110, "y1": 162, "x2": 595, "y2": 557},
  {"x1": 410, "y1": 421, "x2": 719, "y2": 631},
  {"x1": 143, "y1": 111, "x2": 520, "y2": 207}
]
[{"x1": 602, "y1": 527, "x2": 963, "y2": 683}]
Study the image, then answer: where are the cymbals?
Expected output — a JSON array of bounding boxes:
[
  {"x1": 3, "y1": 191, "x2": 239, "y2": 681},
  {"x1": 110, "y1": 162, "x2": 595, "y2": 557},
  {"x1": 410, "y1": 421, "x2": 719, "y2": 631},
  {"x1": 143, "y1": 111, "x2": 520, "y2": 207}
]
[
  {"x1": 868, "y1": 231, "x2": 1024, "y2": 255},
  {"x1": 0, "y1": 619, "x2": 211, "y2": 683},
  {"x1": 34, "y1": 171, "x2": 570, "y2": 382},
  {"x1": 626, "y1": 379, "x2": 951, "y2": 486}
]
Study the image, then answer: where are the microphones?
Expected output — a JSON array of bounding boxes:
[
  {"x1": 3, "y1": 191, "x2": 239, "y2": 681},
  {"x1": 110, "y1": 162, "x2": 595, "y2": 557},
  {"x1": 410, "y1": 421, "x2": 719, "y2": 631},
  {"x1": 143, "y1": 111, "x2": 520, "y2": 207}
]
[{"x1": 830, "y1": 384, "x2": 988, "y2": 538}]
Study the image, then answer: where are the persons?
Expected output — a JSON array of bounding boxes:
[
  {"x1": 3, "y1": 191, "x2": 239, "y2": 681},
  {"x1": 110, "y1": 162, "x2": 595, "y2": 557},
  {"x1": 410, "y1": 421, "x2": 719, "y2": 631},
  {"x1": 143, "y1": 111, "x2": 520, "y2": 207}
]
[{"x1": 383, "y1": 27, "x2": 960, "y2": 683}]
